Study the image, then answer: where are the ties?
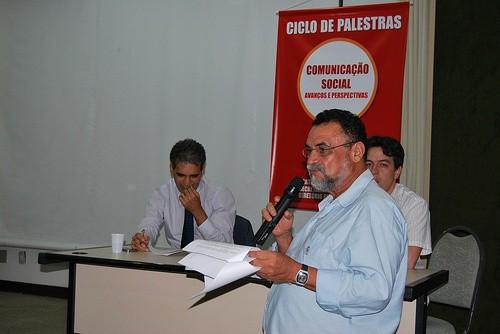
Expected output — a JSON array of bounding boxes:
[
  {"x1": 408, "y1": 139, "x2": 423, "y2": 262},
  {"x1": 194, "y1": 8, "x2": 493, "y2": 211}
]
[{"x1": 180, "y1": 207, "x2": 194, "y2": 249}]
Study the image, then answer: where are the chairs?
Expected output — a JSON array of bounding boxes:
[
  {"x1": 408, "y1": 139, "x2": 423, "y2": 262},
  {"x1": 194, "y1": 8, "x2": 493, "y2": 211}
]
[
  {"x1": 424, "y1": 225, "x2": 484, "y2": 334},
  {"x1": 233, "y1": 214, "x2": 256, "y2": 247}
]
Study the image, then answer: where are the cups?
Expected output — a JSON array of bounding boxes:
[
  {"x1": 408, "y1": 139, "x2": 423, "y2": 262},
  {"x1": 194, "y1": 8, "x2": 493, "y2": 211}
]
[{"x1": 111, "y1": 233, "x2": 125, "y2": 254}]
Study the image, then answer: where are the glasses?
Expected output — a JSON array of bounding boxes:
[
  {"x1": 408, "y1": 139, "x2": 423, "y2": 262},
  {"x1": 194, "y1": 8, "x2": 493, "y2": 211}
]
[{"x1": 302, "y1": 141, "x2": 358, "y2": 158}]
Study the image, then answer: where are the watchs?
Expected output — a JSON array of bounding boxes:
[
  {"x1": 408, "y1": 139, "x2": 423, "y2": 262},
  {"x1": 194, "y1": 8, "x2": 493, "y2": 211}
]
[{"x1": 295, "y1": 264, "x2": 309, "y2": 287}]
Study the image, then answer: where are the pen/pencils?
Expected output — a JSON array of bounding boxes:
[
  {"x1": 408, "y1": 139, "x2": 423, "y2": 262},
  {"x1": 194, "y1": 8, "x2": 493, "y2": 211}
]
[{"x1": 142, "y1": 229, "x2": 144, "y2": 238}]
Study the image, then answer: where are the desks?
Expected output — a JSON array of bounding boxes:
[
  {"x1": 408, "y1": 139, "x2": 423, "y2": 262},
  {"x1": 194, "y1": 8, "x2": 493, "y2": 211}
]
[{"x1": 38, "y1": 247, "x2": 449, "y2": 334}]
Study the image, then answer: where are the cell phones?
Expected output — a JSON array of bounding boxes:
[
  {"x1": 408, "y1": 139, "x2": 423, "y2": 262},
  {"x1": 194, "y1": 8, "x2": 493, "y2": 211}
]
[{"x1": 122, "y1": 247, "x2": 137, "y2": 251}]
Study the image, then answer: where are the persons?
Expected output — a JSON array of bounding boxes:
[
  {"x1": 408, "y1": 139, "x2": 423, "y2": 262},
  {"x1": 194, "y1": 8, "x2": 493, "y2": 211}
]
[
  {"x1": 131, "y1": 139, "x2": 237, "y2": 251},
  {"x1": 365, "y1": 135, "x2": 432, "y2": 307},
  {"x1": 248, "y1": 109, "x2": 408, "y2": 334}
]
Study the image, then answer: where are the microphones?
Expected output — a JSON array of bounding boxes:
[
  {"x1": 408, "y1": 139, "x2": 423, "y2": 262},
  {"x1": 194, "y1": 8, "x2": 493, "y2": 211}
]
[{"x1": 251, "y1": 175, "x2": 305, "y2": 246}]
